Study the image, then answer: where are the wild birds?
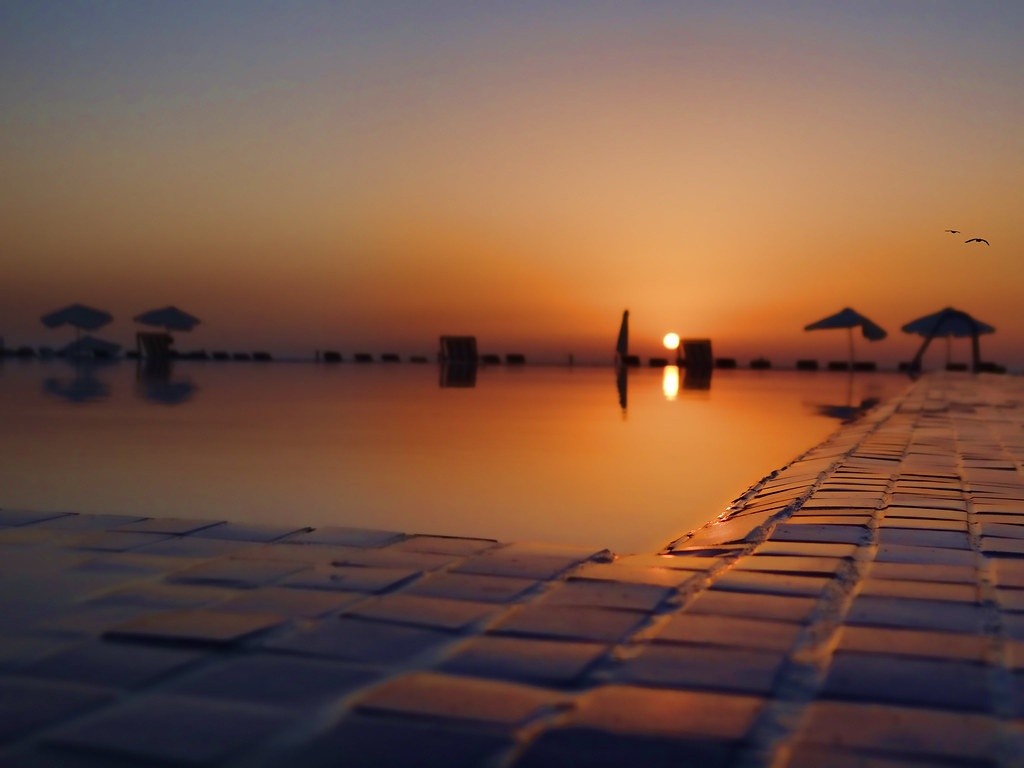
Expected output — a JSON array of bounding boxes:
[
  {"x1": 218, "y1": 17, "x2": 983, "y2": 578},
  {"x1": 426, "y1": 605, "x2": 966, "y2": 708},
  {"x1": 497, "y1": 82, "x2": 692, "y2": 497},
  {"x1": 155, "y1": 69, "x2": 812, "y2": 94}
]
[
  {"x1": 965, "y1": 238, "x2": 991, "y2": 247},
  {"x1": 947, "y1": 229, "x2": 962, "y2": 234}
]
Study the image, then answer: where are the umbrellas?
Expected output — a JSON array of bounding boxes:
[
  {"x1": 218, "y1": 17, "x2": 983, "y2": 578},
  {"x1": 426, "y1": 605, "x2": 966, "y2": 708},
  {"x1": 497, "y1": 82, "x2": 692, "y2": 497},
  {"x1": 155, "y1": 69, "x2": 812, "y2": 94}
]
[
  {"x1": 805, "y1": 306, "x2": 888, "y2": 364},
  {"x1": 134, "y1": 305, "x2": 201, "y2": 337},
  {"x1": 41, "y1": 303, "x2": 113, "y2": 357},
  {"x1": 902, "y1": 307, "x2": 995, "y2": 369}
]
